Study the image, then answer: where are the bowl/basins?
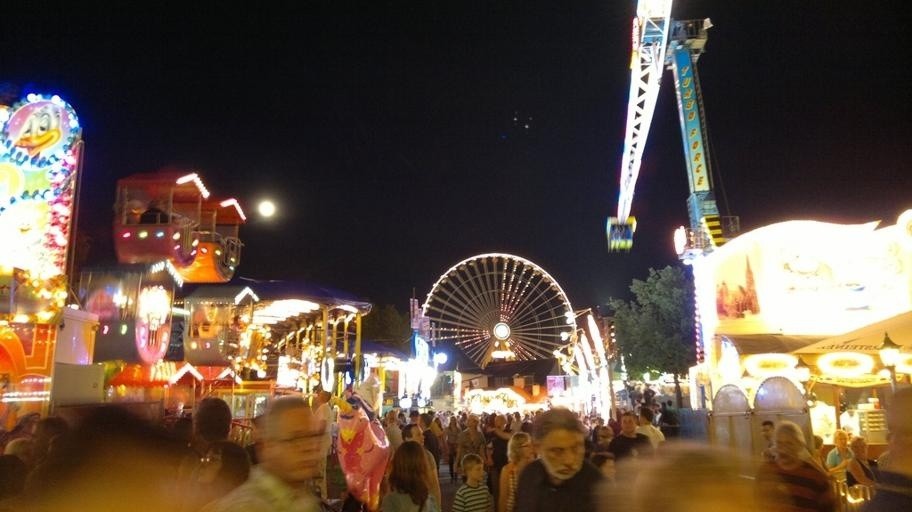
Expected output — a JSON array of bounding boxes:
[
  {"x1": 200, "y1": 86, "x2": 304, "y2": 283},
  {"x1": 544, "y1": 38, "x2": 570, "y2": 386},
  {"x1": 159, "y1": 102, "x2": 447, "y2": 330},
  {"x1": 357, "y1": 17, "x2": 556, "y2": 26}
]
[
  {"x1": 816, "y1": 352, "x2": 872, "y2": 379},
  {"x1": 742, "y1": 352, "x2": 798, "y2": 378}
]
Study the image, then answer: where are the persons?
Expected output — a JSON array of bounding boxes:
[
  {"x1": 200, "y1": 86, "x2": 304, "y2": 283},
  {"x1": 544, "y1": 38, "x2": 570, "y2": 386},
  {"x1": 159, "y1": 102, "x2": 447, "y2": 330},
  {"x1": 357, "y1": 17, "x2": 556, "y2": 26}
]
[
  {"x1": 599, "y1": 444, "x2": 787, "y2": 511},
  {"x1": 0, "y1": 384, "x2": 196, "y2": 511},
  {"x1": 590, "y1": 400, "x2": 679, "y2": 465},
  {"x1": 753, "y1": 390, "x2": 912, "y2": 510},
  {"x1": 170, "y1": 394, "x2": 599, "y2": 511},
  {"x1": 629, "y1": 384, "x2": 655, "y2": 409}
]
